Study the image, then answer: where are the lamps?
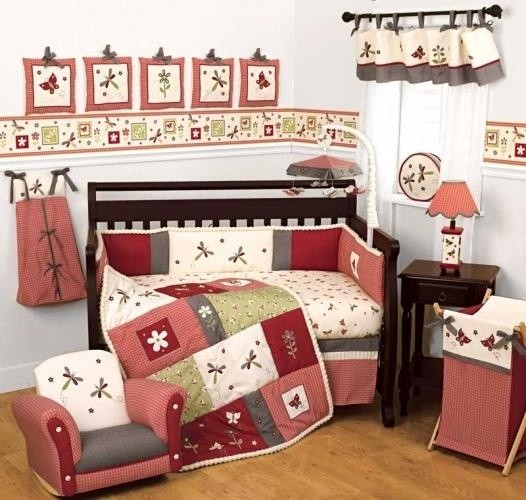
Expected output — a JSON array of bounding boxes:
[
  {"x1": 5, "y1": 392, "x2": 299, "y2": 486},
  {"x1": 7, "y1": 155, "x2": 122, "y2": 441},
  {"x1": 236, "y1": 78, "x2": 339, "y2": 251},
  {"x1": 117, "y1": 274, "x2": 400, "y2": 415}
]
[{"x1": 425, "y1": 179, "x2": 481, "y2": 273}]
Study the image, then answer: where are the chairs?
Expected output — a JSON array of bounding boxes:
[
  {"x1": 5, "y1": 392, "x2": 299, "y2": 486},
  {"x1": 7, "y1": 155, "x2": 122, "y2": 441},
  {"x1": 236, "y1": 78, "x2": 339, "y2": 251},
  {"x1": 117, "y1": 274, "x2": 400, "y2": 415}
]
[{"x1": 11, "y1": 350, "x2": 188, "y2": 497}]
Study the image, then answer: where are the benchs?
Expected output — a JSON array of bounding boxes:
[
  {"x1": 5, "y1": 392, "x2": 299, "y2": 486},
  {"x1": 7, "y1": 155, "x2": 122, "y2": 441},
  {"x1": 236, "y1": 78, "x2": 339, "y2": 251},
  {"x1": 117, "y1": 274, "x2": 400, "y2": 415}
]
[{"x1": 85, "y1": 179, "x2": 400, "y2": 428}]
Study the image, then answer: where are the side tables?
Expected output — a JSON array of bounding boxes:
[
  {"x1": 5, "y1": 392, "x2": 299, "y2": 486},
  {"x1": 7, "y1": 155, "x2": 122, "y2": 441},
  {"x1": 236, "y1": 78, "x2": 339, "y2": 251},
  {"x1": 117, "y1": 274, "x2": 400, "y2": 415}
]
[{"x1": 398, "y1": 259, "x2": 500, "y2": 416}]
